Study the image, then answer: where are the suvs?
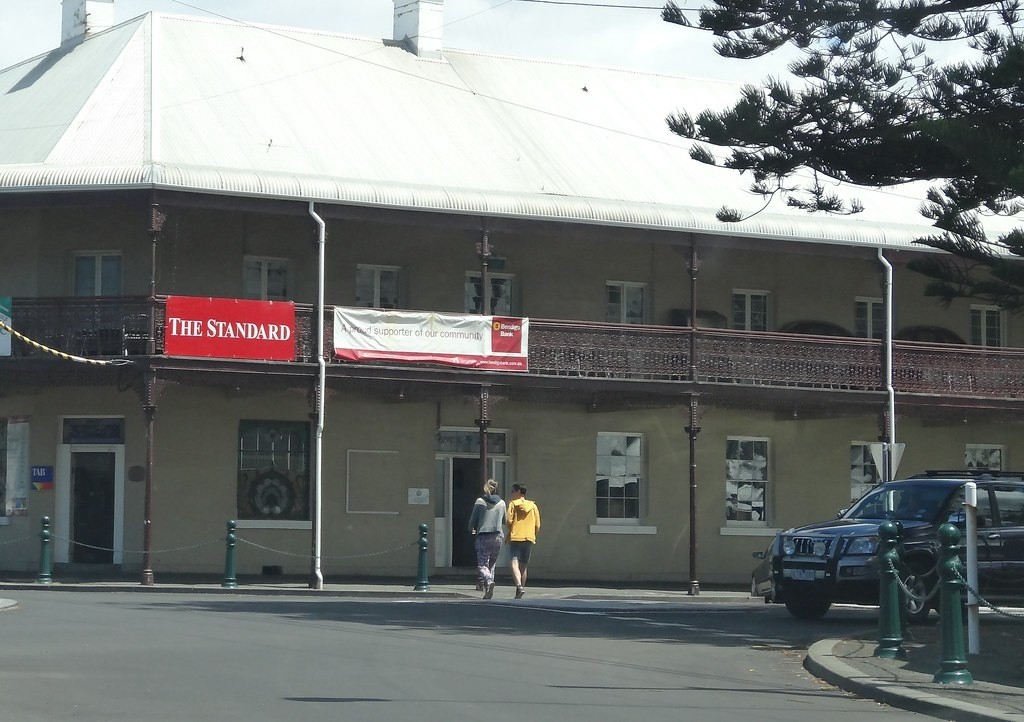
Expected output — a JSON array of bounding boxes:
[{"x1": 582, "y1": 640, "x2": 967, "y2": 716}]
[{"x1": 769, "y1": 469, "x2": 1024, "y2": 617}]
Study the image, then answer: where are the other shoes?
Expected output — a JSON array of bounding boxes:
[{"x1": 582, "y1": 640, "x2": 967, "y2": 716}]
[
  {"x1": 483, "y1": 582, "x2": 495, "y2": 599},
  {"x1": 514, "y1": 587, "x2": 525, "y2": 599}
]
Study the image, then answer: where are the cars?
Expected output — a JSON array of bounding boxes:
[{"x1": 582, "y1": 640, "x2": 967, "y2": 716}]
[{"x1": 751, "y1": 536, "x2": 776, "y2": 596}]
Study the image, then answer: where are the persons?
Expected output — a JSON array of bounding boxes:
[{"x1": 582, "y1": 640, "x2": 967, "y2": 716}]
[
  {"x1": 468, "y1": 479, "x2": 507, "y2": 599},
  {"x1": 505, "y1": 482, "x2": 540, "y2": 599}
]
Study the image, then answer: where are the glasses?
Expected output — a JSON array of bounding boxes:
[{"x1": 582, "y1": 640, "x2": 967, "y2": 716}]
[{"x1": 511, "y1": 490, "x2": 516, "y2": 493}]
